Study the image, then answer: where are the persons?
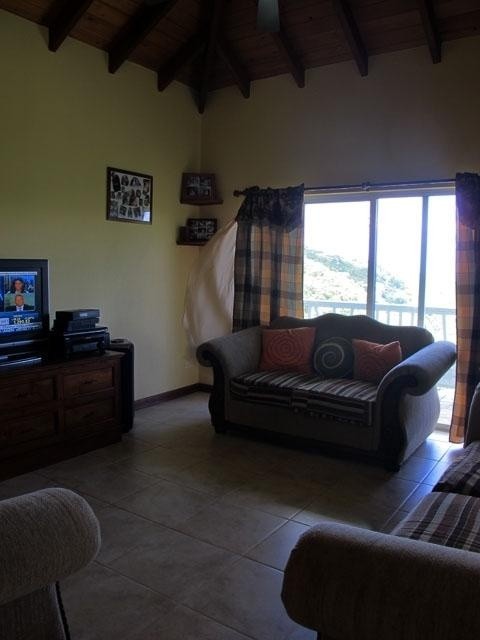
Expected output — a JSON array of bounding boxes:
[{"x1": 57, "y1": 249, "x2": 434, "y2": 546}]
[
  {"x1": 4, "y1": 294, "x2": 35, "y2": 311},
  {"x1": 6, "y1": 278, "x2": 34, "y2": 295}
]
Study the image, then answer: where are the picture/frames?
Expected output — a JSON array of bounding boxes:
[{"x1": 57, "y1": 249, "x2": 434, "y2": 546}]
[
  {"x1": 178, "y1": 217, "x2": 217, "y2": 243},
  {"x1": 180, "y1": 173, "x2": 215, "y2": 200},
  {"x1": 106, "y1": 165, "x2": 153, "y2": 225}
]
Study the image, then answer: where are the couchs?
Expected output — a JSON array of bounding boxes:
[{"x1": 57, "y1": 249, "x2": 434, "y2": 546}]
[{"x1": 195, "y1": 312, "x2": 458, "y2": 472}]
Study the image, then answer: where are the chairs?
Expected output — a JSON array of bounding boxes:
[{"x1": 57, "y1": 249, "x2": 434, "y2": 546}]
[{"x1": 279, "y1": 380, "x2": 479, "y2": 639}]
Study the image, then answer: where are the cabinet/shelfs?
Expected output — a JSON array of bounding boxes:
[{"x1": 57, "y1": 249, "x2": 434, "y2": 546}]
[
  {"x1": 0, "y1": 350, "x2": 127, "y2": 481},
  {"x1": 176, "y1": 200, "x2": 223, "y2": 246}
]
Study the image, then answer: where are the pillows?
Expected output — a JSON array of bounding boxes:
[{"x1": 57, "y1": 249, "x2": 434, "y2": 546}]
[{"x1": 257, "y1": 326, "x2": 403, "y2": 386}]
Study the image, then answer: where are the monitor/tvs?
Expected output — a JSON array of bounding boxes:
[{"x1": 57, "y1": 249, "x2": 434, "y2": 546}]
[{"x1": 0, "y1": 257, "x2": 51, "y2": 367}]
